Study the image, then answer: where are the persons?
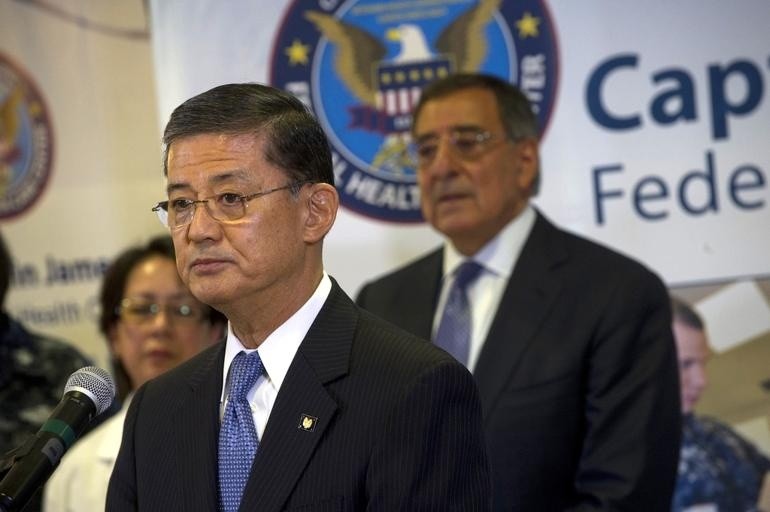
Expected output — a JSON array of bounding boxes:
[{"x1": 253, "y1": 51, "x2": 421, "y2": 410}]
[
  {"x1": 106, "y1": 80, "x2": 487, "y2": 507},
  {"x1": 0, "y1": 225, "x2": 122, "y2": 511},
  {"x1": 352, "y1": 73, "x2": 681, "y2": 511},
  {"x1": 41, "y1": 233, "x2": 229, "y2": 510},
  {"x1": 671, "y1": 297, "x2": 769, "y2": 509}
]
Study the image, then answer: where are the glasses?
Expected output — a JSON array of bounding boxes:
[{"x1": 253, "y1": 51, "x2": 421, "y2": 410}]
[
  {"x1": 152, "y1": 180, "x2": 316, "y2": 230},
  {"x1": 407, "y1": 133, "x2": 511, "y2": 166},
  {"x1": 116, "y1": 297, "x2": 204, "y2": 326}
]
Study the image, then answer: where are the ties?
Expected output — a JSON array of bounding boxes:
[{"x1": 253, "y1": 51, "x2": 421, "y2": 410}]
[
  {"x1": 217, "y1": 350, "x2": 265, "y2": 511},
  {"x1": 436, "y1": 262, "x2": 482, "y2": 367}
]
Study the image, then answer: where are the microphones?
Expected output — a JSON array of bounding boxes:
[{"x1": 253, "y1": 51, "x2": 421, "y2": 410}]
[{"x1": 0, "y1": 366, "x2": 117, "y2": 510}]
